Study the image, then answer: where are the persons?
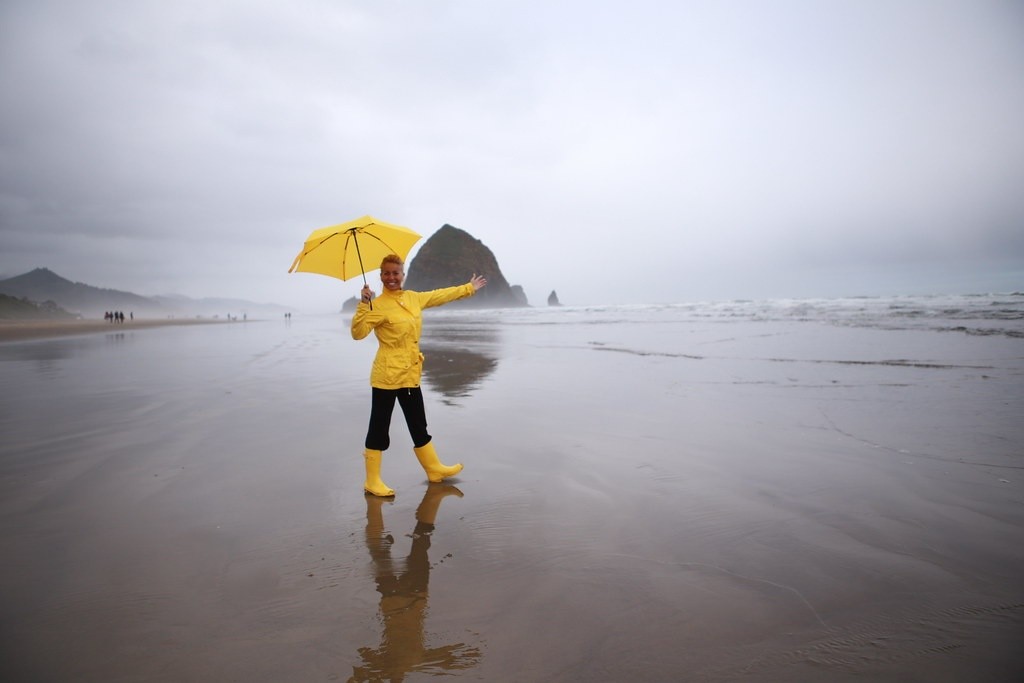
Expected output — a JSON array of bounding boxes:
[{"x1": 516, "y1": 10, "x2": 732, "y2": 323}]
[
  {"x1": 130, "y1": 312, "x2": 133, "y2": 320},
  {"x1": 352, "y1": 254, "x2": 486, "y2": 496},
  {"x1": 104, "y1": 311, "x2": 124, "y2": 323}
]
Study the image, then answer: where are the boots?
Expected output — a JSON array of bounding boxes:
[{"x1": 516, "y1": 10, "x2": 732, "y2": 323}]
[
  {"x1": 414, "y1": 440, "x2": 462, "y2": 482},
  {"x1": 364, "y1": 492, "x2": 394, "y2": 529},
  {"x1": 363, "y1": 447, "x2": 396, "y2": 497},
  {"x1": 416, "y1": 482, "x2": 464, "y2": 524}
]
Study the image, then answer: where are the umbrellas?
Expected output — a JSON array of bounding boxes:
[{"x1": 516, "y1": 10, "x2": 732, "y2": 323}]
[{"x1": 289, "y1": 215, "x2": 422, "y2": 312}]
